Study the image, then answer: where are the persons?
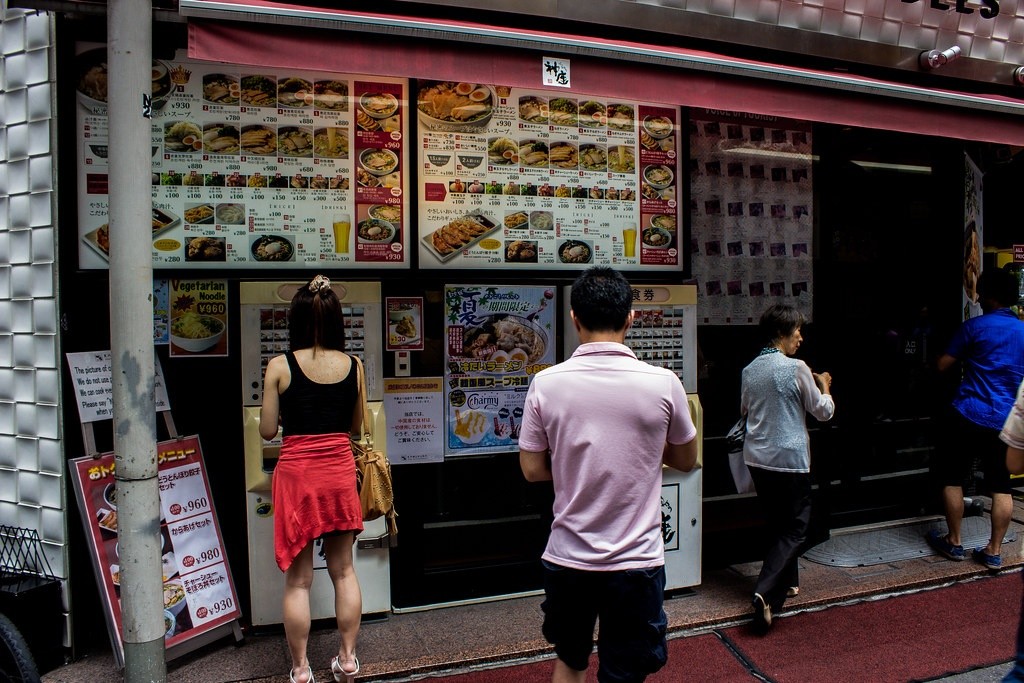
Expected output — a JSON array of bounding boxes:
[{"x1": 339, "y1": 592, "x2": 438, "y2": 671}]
[
  {"x1": 518, "y1": 265, "x2": 698, "y2": 683},
  {"x1": 936, "y1": 267, "x2": 1024, "y2": 569},
  {"x1": 740, "y1": 305, "x2": 834, "y2": 632},
  {"x1": 997, "y1": 376, "x2": 1024, "y2": 683},
  {"x1": 258, "y1": 274, "x2": 369, "y2": 683}
]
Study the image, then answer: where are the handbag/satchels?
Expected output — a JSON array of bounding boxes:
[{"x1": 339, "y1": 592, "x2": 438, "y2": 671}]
[{"x1": 352, "y1": 355, "x2": 398, "y2": 535}]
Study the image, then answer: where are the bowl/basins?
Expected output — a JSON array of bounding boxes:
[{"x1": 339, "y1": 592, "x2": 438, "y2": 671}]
[
  {"x1": 185, "y1": 241, "x2": 226, "y2": 263},
  {"x1": 558, "y1": 240, "x2": 593, "y2": 264},
  {"x1": 641, "y1": 226, "x2": 672, "y2": 250},
  {"x1": 169, "y1": 314, "x2": 226, "y2": 352},
  {"x1": 251, "y1": 235, "x2": 295, "y2": 261},
  {"x1": 164, "y1": 72, "x2": 349, "y2": 161},
  {"x1": 389, "y1": 306, "x2": 416, "y2": 321},
  {"x1": 417, "y1": 82, "x2": 498, "y2": 135},
  {"x1": 530, "y1": 210, "x2": 553, "y2": 231},
  {"x1": 105, "y1": 481, "x2": 186, "y2": 640},
  {"x1": 643, "y1": 115, "x2": 674, "y2": 138},
  {"x1": 488, "y1": 94, "x2": 635, "y2": 175},
  {"x1": 356, "y1": 91, "x2": 401, "y2": 245},
  {"x1": 216, "y1": 202, "x2": 245, "y2": 225},
  {"x1": 450, "y1": 314, "x2": 551, "y2": 374},
  {"x1": 643, "y1": 164, "x2": 674, "y2": 189},
  {"x1": 504, "y1": 244, "x2": 538, "y2": 263},
  {"x1": 650, "y1": 214, "x2": 676, "y2": 237},
  {"x1": 75, "y1": 47, "x2": 178, "y2": 115}
]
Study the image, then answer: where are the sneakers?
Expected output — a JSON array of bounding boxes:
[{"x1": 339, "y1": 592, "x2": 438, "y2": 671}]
[
  {"x1": 935, "y1": 532, "x2": 967, "y2": 561},
  {"x1": 973, "y1": 546, "x2": 1003, "y2": 569}
]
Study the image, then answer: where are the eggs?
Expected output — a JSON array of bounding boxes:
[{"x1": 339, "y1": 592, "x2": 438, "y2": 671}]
[
  {"x1": 228, "y1": 82, "x2": 241, "y2": 98},
  {"x1": 489, "y1": 348, "x2": 528, "y2": 371},
  {"x1": 455, "y1": 82, "x2": 490, "y2": 102},
  {"x1": 182, "y1": 134, "x2": 202, "y2": 151},
  {"x1": 503, "y1": 149, "x2": 518, "y2": 164},
  {"x1": 644, "y1": 117, "x2": 670, "y2": 134},
  {"x1": 650, "y1": 234, "x2": 661, "y2": 242},
  {"x1": 294, "y1": 89, "x2": 313, "y2": 105},
  {"x1": 520, "y1": 147, "x2": 531, "y2": 156},
  {"x1": 539, "y1": 105, "x2": 549, "y2": 118},
  {"x1": 592, "y1": 111, "x2": 606, "y2": 126},
  {"x1": 367, "y1": 226, "x2": 381, "y2": 235},
  {"x1": 569, "y1": 246, "x2": 582, "y2": 257}
]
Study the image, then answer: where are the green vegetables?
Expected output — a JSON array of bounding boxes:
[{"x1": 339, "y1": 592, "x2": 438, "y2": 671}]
[
  {"x1": 241, "y1": 75, "x2": 276, "y2": 104},
  {"x1": 200, "y1": 316, "x2": 222, "y2": 334},
  {"x1": 617, "y1": 105, "x2": 634, "y2": 121},
  {"x1": 532, "y1": 142, "x2": 548, "y2": 154},
  {"x1": 549, "y1": 98, "x2": 577, "y2": 117},
  {"x1": 326, "y1": 81, "x2": 348, "y2": 110},
  {"x1": 161, "y1": 172, "x2": 182, "y2": 185},
  {"x1": 205, "y1": 173, "x2": 225, "y2": 186},
  {"x1": 580, "y1": 101, "x2": 606, "y2": 115}
]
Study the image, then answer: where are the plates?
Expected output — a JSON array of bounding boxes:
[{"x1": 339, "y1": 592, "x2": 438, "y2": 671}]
[
  {"x1": 504, "y1": 210, "x2": 529, "y2": 230},
  {"x1": 82, "y1": 200, "x2": 180, "y2": 261},
  {"x1": 185, "y1": 203, "x2": 215, "y2": 225},
  {"x1": 420, "y1": 206, "x2": 501, "y2": 262},
  {"x1": 390, "y1": 324, "x2": 420, "y2": 346}
]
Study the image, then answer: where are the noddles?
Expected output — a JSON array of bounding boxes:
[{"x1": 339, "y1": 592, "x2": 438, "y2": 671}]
[
  {"x1": 362, "y1": 94, "x2": 396, "y2": 114},
  {"x1": 646, "y1": 167, "x2": 671, "y2": 185},
  {"x1": 169, "y1": 312, "x2": 223, "y2": 339},
  {"x1": 518, "y1": 141, "x2": 548, "y2": 166},
  {"x1": 579, "y1": 148, "x2": 608, "y2": 171},
  {"x1": 362, "y1": 152, "x2": 396, "y2": 171},
  {"x1": 416, "y1": 80, "x2": 494, "y2": 123},
  {"x1": 314, "y1": 127, "x2": 349, "y2": 157},
  {"x1": 371, "y1": 204, "x2": 401, "y2": 223},
  {"x1": 609, "y1": 145, "x2": 635, "y2": 172},
  {"x1": 549, "y1": 145, "x2": 579, "y2": 168}
]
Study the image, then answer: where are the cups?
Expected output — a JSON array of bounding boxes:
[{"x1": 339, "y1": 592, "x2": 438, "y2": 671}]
[
  {"x1": 333, "y1": 213, "x2": 351, "y2": 254},
  {"x1": 622, "y1": 222, "x2": 637, "y2": 258}
]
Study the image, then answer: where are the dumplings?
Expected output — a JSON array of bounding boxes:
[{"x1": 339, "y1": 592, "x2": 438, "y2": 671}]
[{"x1": 432, "y1": 219, "x2": 486, "y2": 254}]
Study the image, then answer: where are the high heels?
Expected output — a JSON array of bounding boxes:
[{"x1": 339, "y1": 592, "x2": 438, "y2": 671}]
[
  {"x1": 289, "y1": 666, "x2": 314, "y2": 683},
  {"x1": 331, "y1": 654, "x2": 360, "y2": 683}
]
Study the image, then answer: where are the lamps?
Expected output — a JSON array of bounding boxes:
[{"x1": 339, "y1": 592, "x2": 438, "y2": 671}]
[
  {"x1": 921, "y1": 45, "x2": 961, "y2": 73},
  {"x1": 1014, "y1": 66, "x2": 1024, "y2": 82}
]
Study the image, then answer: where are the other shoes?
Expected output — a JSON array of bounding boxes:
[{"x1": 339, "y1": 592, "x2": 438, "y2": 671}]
[
  {"x1": 785, "y1": 586, "x2": 800, "y2": 597},
  {"x1": 750, "y1": 593, "x2": 772, "y2": 631}
]
